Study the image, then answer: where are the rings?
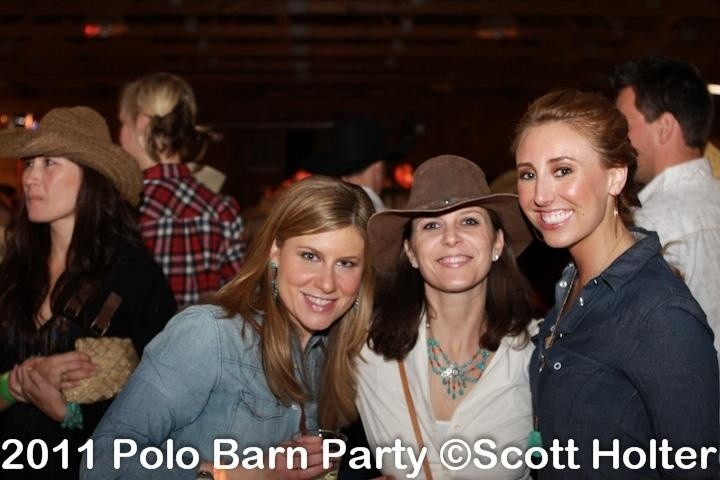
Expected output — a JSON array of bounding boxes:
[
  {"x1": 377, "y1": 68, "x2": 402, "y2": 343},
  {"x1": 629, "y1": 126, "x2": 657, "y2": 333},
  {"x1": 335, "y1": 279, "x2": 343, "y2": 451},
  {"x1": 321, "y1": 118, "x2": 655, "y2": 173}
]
[{"x1": 60, "y1": 370, "x2": 69, "y2": 385}]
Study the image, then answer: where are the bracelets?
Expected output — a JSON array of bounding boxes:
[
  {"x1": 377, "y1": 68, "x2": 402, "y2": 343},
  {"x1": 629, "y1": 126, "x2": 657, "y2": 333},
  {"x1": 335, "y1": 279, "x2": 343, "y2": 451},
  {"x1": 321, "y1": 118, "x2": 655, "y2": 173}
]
[
  {"x1": 59, "y1": 402, "x2": 83, "y2": 430},
  {"x1": 0, "y1": 369, "x2": 17, "y2": 406}
]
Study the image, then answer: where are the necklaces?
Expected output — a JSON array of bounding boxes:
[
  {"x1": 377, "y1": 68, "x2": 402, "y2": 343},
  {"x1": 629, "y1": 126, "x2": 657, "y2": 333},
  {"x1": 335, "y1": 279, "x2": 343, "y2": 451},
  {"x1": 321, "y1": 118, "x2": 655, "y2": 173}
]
[{"x1": 425, "y1": 322, "x2": 493, "y2": 401}]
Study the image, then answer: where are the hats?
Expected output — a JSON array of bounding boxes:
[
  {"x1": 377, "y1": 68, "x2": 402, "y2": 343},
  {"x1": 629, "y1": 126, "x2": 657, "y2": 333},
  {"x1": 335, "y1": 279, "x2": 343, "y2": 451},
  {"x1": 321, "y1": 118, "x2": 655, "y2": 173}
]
[
  {"x1": 368, "y1": 154, "x2": 532, "y2": 275},
  {"x1": 0, "y1": 105, "x2": 140, "y2": 207}
]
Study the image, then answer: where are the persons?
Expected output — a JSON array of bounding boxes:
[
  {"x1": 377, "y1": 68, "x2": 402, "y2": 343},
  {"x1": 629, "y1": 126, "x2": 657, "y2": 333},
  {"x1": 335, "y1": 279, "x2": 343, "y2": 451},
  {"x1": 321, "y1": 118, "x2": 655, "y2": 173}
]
[
  {"x1": 514, "y1": 91, "x2": 720, "y2": 479},
  {"x1": 79, "y1": 176, "x2": 377, "y2": 479},
  {"x1": 120, "y1": 71, "x2": 248, "y2": 313},
  {"x1": 349, "y1": 153, "x2": 535, "y2": 479},
  {"x1": 0, "y1": 109, "x2": 178, "y2": 479},
  {"x1": 615, "y1": 63, "x2": 714, "y2": 356}
]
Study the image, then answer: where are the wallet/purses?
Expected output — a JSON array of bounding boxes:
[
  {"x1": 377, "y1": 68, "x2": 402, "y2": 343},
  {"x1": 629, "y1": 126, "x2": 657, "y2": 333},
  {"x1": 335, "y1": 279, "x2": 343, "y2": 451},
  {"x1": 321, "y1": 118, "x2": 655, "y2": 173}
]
[{"x1": 62, "y1": 336, "x2": 138, "y2": 404}]
